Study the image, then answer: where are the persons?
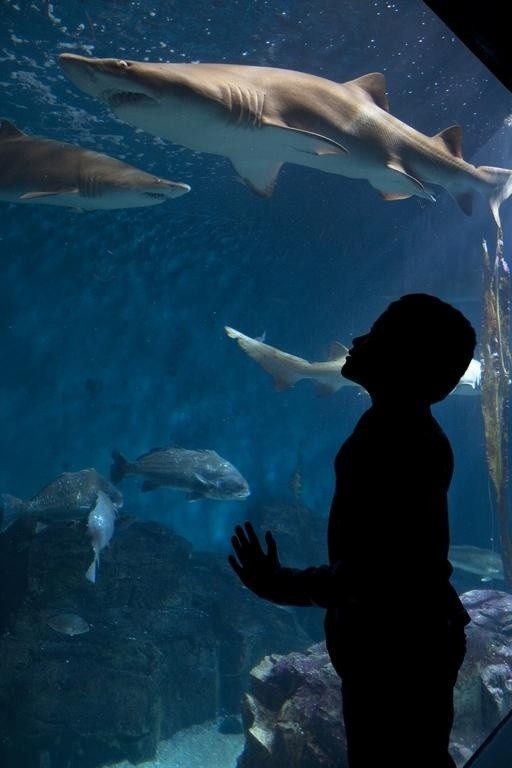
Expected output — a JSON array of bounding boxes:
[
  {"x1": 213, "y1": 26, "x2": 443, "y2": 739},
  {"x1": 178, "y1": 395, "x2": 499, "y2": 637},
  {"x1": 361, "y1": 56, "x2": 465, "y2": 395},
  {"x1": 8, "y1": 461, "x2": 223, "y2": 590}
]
[{"x1": 225, "y1": 292, "x2": 479, "y2": 767}]
